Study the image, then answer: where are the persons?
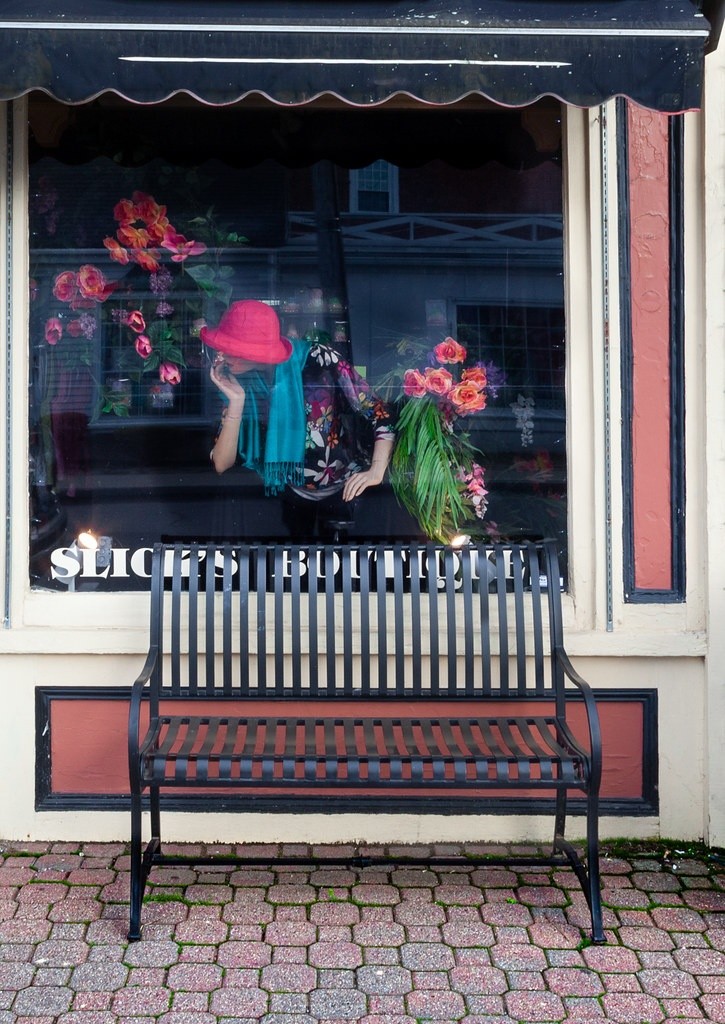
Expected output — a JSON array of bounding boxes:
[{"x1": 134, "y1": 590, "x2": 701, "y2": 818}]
[
  {"x1": 201, "y1": 299, "x2": 444, "y2": 593},
  {"x1": 42, "y1": 354, "x2": 98, "y2": 497}
]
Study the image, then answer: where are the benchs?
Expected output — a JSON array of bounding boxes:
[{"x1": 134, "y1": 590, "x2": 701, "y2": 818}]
[{"x1": 128, "y1": 543, "x2": 609, "y2": 946}]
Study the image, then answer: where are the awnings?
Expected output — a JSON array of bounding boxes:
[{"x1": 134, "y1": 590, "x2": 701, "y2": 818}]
[{"x1": 0, "y1": 0, "x2": 725, "y2": 115}]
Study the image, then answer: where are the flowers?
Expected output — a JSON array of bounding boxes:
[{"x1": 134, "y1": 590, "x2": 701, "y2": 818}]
[{"x1": 30, "y1": 179, "x2": 564, "y2": 548}]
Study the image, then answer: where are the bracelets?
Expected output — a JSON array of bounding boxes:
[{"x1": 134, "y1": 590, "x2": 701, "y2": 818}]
[
  {"x1": 373, "y1": 460, "x2": 381, "y2": 462},
  {"x1": 226, "y1": 416, "x2": 242, "y2": 420}
]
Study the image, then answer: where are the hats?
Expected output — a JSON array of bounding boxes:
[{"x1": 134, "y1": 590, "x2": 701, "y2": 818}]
[{"x1": 199, "y1": 300, "x2": 292, "y2": 365}]
